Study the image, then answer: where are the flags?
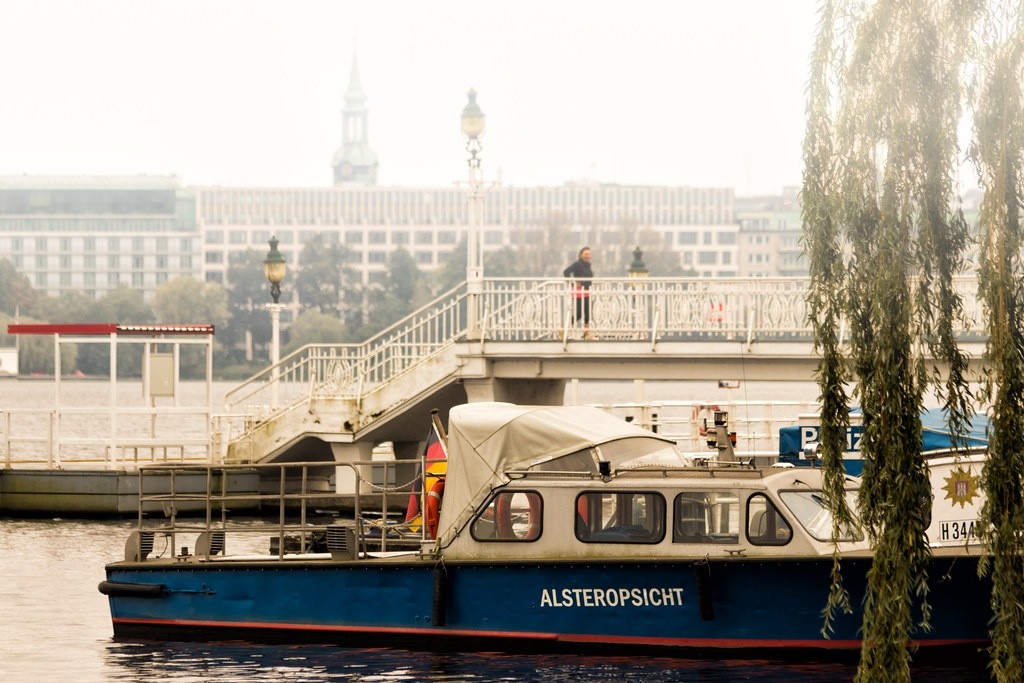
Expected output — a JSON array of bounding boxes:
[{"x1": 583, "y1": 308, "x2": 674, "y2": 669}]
[{"x1": 405, "y1": 425, "x2": 448, "y2": 532}]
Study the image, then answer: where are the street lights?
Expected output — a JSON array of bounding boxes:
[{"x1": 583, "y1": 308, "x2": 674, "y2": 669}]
[
  {"x1": 459, "y1": 87, "x2": 487, "y2": 338},
  {"x1": 264, "y1": 235, "x2": 287, "y2": 411},
  {"x1": 626, "y1": 246, "x2": 650, "y2": 403}
]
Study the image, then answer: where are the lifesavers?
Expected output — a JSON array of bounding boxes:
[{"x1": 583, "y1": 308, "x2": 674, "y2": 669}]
[
  {"x1": 497, "y1": 492, "x2": 541, "y2": 540},
  {"x1": 425, "y1": 481, "x2": 445, "y2": 540},
  {"x1": 692, "y1": 406, "x2": 720, "y2": 436}
]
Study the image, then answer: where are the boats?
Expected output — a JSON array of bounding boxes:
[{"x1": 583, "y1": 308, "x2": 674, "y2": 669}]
[{"x1": 98, "y1": 402, "x2": 997, "y2": 662}]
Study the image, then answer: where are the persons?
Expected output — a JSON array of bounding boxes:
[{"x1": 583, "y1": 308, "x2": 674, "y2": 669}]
[{"x1": 563, "y1": 247, "x2": 598, "y2": 340}]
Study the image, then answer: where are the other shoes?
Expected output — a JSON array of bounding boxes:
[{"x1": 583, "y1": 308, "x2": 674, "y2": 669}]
[
  {"x1": 580, "y1": 324, "x2": 600, "y2": 340},
  {"x1": 560, "y1": 327, "x2": 570, "y2": 340}
]
